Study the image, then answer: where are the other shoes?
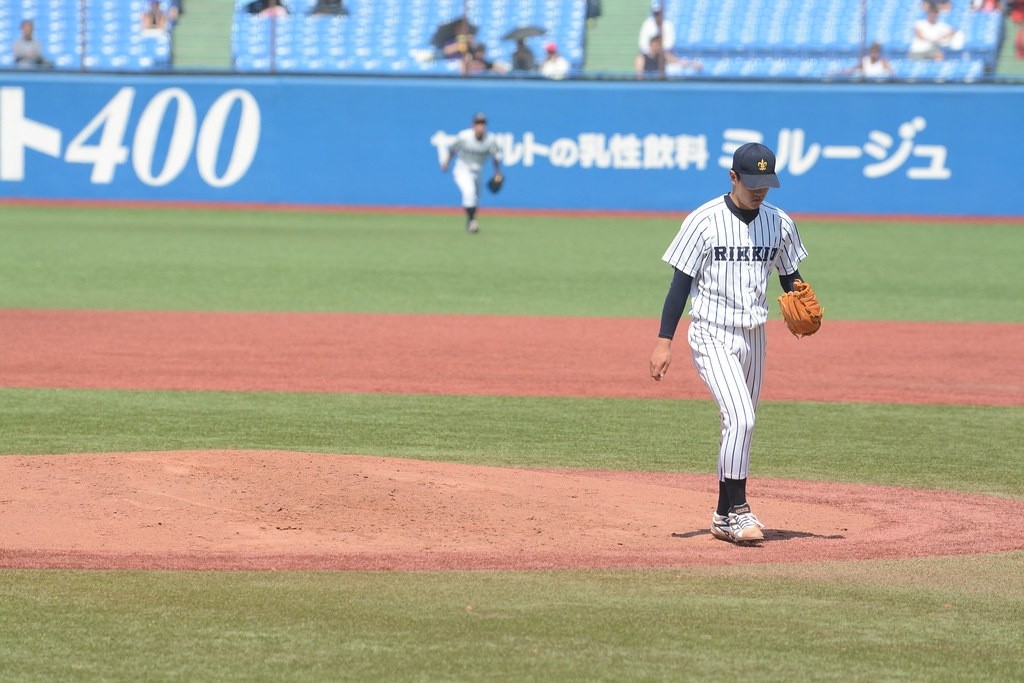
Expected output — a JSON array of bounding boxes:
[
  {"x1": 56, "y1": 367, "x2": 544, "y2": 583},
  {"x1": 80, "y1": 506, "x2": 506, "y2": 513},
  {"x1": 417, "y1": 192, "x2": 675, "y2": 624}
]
[{"x1": 468, "y1": 220, "x2": 476, "y2": 232}]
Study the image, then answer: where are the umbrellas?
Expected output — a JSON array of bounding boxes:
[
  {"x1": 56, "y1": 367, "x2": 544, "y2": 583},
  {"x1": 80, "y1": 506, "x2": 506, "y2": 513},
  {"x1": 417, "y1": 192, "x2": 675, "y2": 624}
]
[
  {"x1": 431, "y1": 17, "x2": 478, "y2": 47},
  {"x1": 500, "y1": 28, "x2": 548, "y2": 39}
]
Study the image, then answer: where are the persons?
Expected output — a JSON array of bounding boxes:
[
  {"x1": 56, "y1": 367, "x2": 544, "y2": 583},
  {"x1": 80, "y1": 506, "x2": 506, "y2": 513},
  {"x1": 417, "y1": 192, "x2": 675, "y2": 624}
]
[
  {"x1": 13, "y1": 19, "x2": 54, "y2": 67},
  {"x1": 442, "y1": 113, "x2": 504, "y2": 232},
  {"x1": 649, "y1": 143, "x2": 825, "y2": 542},
  {"x1": 438, "y1": 21, "x2": 571, "y2": 80},
  {"x1": 844, "y1": 0, "x2": 1024, "y2": 84},
  {"x1": 639, "y1": 0, "x2": 676, "y2": 56},
  {"x1": 140, "y1": 0, "x2": 351, "y2": 32},
  {"x1": 635, "y1": 34, "x2": 704, "y2": 81}
]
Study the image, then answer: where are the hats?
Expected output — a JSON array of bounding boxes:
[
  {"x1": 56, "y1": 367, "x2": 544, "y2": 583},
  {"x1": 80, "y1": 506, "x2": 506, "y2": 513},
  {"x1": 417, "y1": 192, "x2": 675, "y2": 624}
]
[
  {"x1": 733, "y1": 143, "x2": 780, "y2": 189},
  {"x1": 475, "y1": 114, "x2": 485, "y2": 123}
]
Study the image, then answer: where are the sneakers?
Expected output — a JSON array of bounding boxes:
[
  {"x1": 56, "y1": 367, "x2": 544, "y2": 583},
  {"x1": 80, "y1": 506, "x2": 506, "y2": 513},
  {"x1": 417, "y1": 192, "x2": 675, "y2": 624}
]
[
  {"x1": 711, "y1": 511, "x2": 731, "y2": 540},
  {"x1": 728, "y1": 502, "x2": 765, "y2": 542}
]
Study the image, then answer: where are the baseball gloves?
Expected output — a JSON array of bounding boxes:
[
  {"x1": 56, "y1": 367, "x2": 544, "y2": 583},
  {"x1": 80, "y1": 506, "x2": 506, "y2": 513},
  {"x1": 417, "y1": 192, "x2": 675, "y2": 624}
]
[
  {"x1": 777, "y1": 279, "x2": 824, "y2": 337},
  {"x1": 487, "y1": 173, "x2": 504, "y2": 193}
]
